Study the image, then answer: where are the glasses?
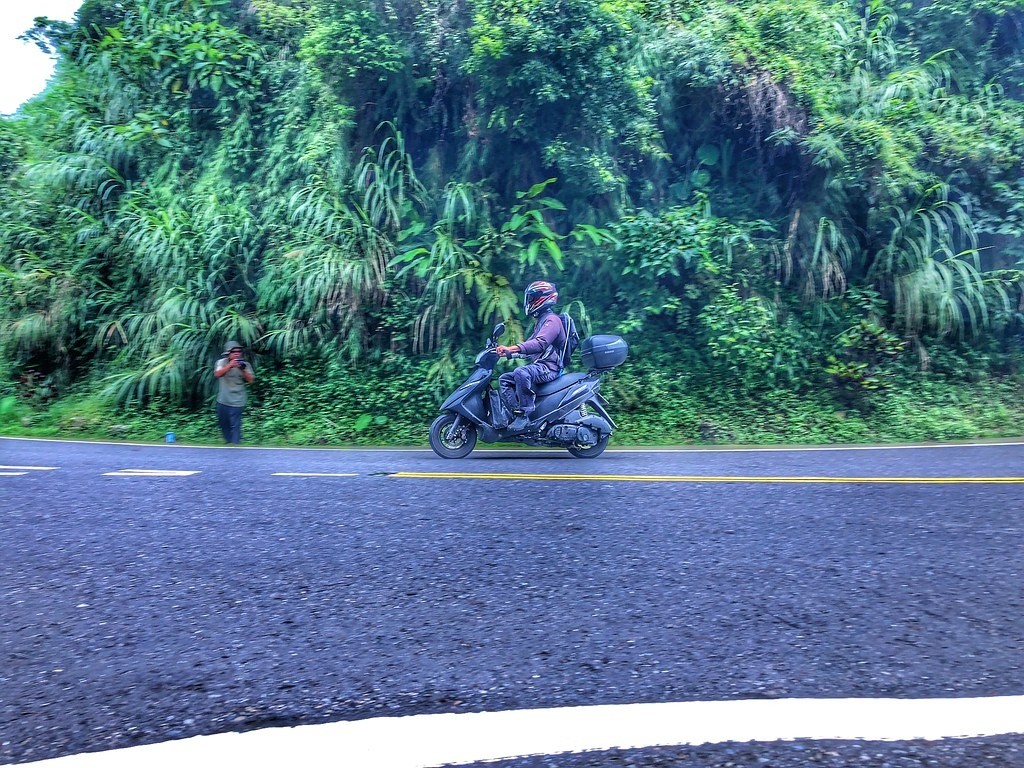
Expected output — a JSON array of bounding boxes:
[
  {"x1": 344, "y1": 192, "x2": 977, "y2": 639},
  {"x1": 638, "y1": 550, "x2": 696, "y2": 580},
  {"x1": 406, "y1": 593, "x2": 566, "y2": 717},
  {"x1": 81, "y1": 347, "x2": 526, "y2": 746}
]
[{"x1": 230, "y1": 351, "x2": 242, "y2": 353}]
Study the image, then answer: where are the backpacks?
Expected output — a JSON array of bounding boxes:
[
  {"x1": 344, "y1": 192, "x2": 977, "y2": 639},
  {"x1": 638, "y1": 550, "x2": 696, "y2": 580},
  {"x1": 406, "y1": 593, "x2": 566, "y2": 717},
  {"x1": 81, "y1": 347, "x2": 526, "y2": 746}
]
[{"x1": 536, "y1": 312, "x2": 578, "y2": 370}]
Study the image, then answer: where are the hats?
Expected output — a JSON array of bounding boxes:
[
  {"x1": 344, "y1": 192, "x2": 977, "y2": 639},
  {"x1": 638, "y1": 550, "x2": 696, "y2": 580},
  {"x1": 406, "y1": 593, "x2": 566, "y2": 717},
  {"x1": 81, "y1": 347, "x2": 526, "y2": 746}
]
[{"x1": 221, "y1": 341, "x2": 245, "y2": 355}]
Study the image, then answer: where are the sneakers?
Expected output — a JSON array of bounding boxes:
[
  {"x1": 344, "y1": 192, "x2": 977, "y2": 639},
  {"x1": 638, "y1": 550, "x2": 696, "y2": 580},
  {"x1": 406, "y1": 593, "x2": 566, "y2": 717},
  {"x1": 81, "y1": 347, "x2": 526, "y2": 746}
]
[{"x1": 507, "y1": 417, "x2": 531, "y2": 431}]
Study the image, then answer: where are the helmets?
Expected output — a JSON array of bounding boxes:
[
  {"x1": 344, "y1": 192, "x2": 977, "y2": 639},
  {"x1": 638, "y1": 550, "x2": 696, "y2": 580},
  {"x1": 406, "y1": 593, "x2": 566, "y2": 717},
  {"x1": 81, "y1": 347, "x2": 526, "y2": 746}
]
[{"x1": 524, "y1": 281, "x2": 559, "y2": 316}]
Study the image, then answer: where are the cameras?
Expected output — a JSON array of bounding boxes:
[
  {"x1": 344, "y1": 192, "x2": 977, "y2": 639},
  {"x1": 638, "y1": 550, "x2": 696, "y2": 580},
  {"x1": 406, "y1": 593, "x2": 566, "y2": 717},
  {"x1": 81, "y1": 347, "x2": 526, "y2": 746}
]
[{"x1": 235, "y1": 359, "x2": 247, "y2": 371}]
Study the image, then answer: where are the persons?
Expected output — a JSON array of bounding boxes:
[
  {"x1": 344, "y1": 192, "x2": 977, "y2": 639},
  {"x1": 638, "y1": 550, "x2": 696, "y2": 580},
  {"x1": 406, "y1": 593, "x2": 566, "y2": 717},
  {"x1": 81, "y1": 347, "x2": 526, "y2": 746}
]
[
  {"x1": 213, "y1": 341, "x2": 255, "y2": 444},
  {"x1": 496, "y1": 281, "x2": 571, "y2": 432}
]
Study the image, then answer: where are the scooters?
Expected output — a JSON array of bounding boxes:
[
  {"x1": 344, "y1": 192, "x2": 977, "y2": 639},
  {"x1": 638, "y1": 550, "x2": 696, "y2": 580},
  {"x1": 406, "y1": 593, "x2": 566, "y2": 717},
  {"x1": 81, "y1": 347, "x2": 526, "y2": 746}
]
[{"x1": 429, "y1": 323, "x2": 629, "y2": 459}]
